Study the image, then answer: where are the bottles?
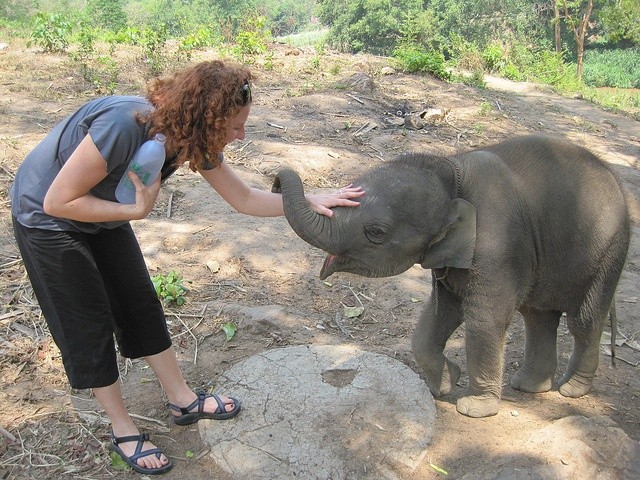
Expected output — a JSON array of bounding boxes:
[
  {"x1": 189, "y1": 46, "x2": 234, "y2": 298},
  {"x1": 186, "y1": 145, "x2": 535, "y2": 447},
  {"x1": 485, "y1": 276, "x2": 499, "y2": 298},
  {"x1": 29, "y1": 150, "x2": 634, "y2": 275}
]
[{"x1": 114, "y1": 133, "x2": 167, "y2": 204}]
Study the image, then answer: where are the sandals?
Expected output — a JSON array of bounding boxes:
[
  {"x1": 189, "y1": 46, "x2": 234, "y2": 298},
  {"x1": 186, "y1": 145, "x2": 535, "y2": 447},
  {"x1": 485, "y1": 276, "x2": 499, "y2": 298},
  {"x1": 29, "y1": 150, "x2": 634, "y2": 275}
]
[
  {"x1": 168, "y1": 391, "x2": 241, "y2": 426},
  {"x1": 110, "y1": 429, "x2": 173, "y2": 475}
]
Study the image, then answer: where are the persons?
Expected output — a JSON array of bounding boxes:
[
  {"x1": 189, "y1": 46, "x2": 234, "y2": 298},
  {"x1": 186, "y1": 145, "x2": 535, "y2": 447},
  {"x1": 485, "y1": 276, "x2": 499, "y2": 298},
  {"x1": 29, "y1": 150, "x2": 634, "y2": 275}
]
[{"x1": 10, "y1": 61, "x2": 365, "y2": 474}]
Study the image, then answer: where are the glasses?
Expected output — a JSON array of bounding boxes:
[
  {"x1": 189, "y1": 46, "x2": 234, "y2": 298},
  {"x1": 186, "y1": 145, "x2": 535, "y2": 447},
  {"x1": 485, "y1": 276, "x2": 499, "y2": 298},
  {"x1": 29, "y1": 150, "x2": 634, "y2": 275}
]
[{"x1": 235, "y1": 83, "x2": 252, "y2": 105}]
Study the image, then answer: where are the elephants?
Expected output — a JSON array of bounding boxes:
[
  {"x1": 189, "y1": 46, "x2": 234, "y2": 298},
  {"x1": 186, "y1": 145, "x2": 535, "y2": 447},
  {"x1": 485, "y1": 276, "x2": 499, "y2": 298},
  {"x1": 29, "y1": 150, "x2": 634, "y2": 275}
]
[{"x1": 271, "y1": 133, "x2": 632, "y2": 418}]
